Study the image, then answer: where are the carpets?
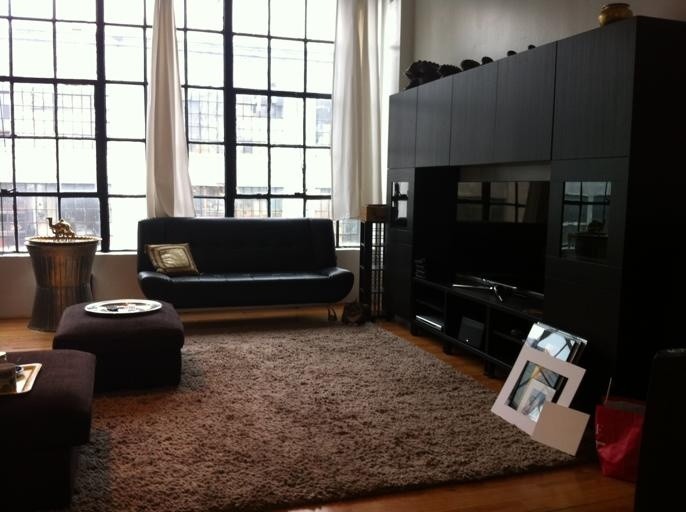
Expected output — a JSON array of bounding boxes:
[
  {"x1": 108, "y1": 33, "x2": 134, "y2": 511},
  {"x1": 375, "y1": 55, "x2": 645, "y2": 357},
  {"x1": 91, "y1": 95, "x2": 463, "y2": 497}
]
[{"x1": 68, "y1": 321, "x2": 587, "y2": 512}]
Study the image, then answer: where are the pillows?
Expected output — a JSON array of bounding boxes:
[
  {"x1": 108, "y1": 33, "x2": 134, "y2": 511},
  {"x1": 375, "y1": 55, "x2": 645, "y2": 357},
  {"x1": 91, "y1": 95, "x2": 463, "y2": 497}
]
[{"x1": 144, "y1": 243, "x2": 199, "y2": 274}]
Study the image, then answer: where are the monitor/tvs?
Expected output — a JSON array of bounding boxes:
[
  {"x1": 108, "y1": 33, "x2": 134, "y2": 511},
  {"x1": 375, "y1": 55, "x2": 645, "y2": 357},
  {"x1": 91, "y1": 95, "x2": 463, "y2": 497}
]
[{"x1": 452, "y1": 179, "x2": 550, "y2": 304}]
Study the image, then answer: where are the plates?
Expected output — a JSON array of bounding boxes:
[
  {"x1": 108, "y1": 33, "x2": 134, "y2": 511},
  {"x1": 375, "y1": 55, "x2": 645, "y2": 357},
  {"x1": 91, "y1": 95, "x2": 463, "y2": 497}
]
[{"x1": 84, "y1": 299, "x2": 163, "y2": 314}]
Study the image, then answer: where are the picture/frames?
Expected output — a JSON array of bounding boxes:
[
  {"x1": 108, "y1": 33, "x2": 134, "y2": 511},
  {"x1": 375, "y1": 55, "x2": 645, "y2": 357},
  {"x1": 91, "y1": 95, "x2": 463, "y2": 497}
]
[
  {"x1": 490, "y1": 345, "x2": 587, "y2": 437},
  {"x1": 517, "y1": 378, "x2": 557, "y2": 415}
]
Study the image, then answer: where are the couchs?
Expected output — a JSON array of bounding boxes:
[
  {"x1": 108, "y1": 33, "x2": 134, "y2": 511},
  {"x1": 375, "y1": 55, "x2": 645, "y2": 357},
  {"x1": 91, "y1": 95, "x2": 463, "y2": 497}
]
[{"x1": 137, "y1": 217, "x2": 354, "y2": 322}]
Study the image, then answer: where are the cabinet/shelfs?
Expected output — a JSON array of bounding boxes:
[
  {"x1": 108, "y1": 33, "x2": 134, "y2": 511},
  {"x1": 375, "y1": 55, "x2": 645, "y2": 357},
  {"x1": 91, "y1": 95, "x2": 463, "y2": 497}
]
[
  {"x1": 410, "y1": 274, "x2": 545, "y2": 378},
  {"x1": 359, "y1": 220, "x2": 391, "y2": 319}
]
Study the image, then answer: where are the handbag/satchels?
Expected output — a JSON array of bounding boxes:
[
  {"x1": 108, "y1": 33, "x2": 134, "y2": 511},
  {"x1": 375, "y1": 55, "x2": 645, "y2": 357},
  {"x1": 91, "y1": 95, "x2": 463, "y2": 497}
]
[{"x1": 594, "y1": 377, "x2": 647, "y2": 482}]
